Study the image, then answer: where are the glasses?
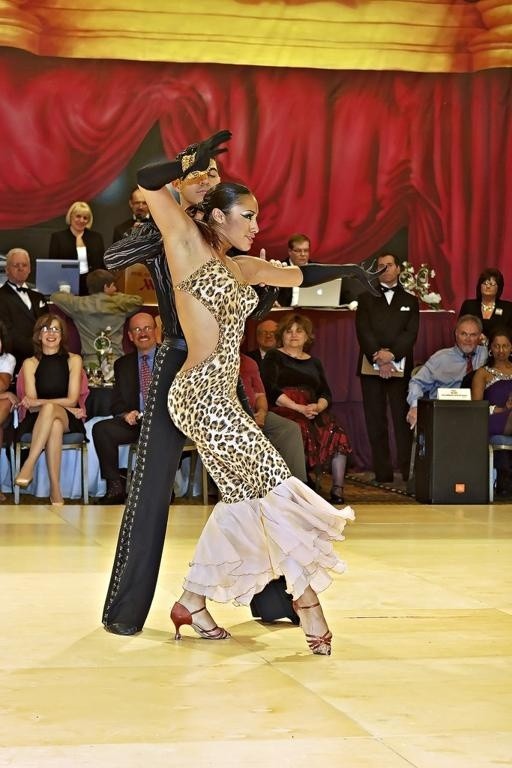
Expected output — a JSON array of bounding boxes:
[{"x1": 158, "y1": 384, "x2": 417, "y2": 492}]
[
  {"x1": 40, "y1": 327, "x2": 62, "y2": 333},
  {"x1": 482, "y1": 282, "x2": 498, "y2": 286},
  {"x1": 291, "y1": 248, "x2": 309, "y2": 255},
  {"x1": 132, "y1": 326, "x2": 156, "y2": 333}
]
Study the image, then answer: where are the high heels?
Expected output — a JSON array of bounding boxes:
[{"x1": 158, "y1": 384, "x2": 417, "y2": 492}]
[
  {"x1": 171, "y1": 603, "x2": 232, "y2": 641},
  {"x1": 294, "y1": 600, "x2": 331, "y2": 656}
]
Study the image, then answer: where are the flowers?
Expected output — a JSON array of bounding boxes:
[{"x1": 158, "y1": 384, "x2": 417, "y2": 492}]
[{"x1": 402, "y1": 260, "x2": 443, "y2": 311}]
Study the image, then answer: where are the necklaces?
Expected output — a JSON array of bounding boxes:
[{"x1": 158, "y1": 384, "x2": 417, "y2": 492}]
[{"x1": 481, "y1": 302, "x2": 495, "y2": 313}]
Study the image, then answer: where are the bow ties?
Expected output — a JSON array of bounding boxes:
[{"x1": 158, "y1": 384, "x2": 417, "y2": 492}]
[
  {"x1": 8, "y1": 281, "x2": 28, "y2": 293},
  {"x1": 382, "y1": 285, "x2": 398, "y2": 293}
]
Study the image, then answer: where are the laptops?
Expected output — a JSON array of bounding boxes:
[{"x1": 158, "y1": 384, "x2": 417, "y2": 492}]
[
  {"x1": 292, "y1": 264, "x2": 342, "y2": 310},
  {"x1": 37, "y1": 259, "x2": 79, "y2": 295}
]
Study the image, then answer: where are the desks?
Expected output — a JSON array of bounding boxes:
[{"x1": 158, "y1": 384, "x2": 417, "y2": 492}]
[{"x1": 38, "y1": 301, "x2": 457, "y2": 400}]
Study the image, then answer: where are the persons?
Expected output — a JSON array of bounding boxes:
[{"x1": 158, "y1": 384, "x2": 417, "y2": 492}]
[
  {"x1": 13, "y1": 312, "x2": 87, "y2": 506},
  {"x1": 100, "y1": 143, "x2": 300, "y2": 634},
  {"x1": 134, "y1": 130, "x2": 388, "y2": 657},
  {"x1": 261, "y1": 313, "x2": 353, "y2": 504},
  {"x1": 471, "y1": 333, "x2": 512, "y2": 502},
  {"x1": 456, "y1": 268, "x2": 512, "y2": 339},
  {"x1": 236, "y1": 322, "x2": 306, "y2": 483},
  {"x1": 112, "y1": 185, "x2": 163, "y2": 243},
  {"x1": 355, "y1": 252, "x2": 419, "y2": 484},
  {"x1": 0, "y1": 323, "x2": 21, "y2": 501},
  {"x1": 245, "y1": 318, "x2": 276, "y2": 373},
  {"x1": 274, "y1": 234, "x2": 353, "y2": 309},
  {"x1": 50, "y1": 269, "x2": 142, "y2": 370},
  {"x1": 0, "y1": 247, "x2": 50, "y2": 386},
  {"x1": 47, "y1": 200, "x2": 104, "y2": 297},
  {"x1": 91, "y1": 310, "x2": 189, "y2": 505},
  {"x1": 405, "y1": 315, "x2": 486, "y2": 430}
]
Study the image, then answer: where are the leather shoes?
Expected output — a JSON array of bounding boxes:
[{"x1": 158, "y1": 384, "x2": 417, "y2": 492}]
[
  {"x1": 108, "y1": 622, "x2": 138, "y2": 634},
  {"x1": 15, "y1": 474, "x2": 33, "y2": 486},
  {"x1": 94, "y1": 480, "x2": 125, "y2": 505},
  {"x1": 50, "y1": 494, "x2": 66, "y2": 506},
  {"x1": 330, "y1": 484, "x2": 345, "y2": 504},
  {"x1": 262, "y1": 604, "x2": 300, "y2": 625}
]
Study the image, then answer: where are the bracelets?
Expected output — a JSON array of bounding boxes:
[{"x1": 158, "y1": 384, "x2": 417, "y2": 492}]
[{"x1": 489, "y1": 404, "x2": 496, "y2": 415}]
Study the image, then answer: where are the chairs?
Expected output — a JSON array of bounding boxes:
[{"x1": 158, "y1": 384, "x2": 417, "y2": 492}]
[
  {"x1": 2, "y1": 408, "x2": 18, "y2": 495},
  {"x1": 12, "y1": 431, "x2": 90, "y2": 504},
  {"x1": 487, "y1": 433, "x2": 512, "y2": 504},
  {"x1": 125, "y1": 437, "x2": 209, "y2": 506},
  {"x1": 409, "y1": 366, "x2": 424, "y2": 480}
]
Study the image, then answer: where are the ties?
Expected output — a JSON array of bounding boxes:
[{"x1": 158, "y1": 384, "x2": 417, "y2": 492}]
[
  {"x1": 141, "y1": 356, "x2": 151, "y2": 405},
  {"x1": 466, "y1": 354, "x2": 474, "y2": 374}
]
